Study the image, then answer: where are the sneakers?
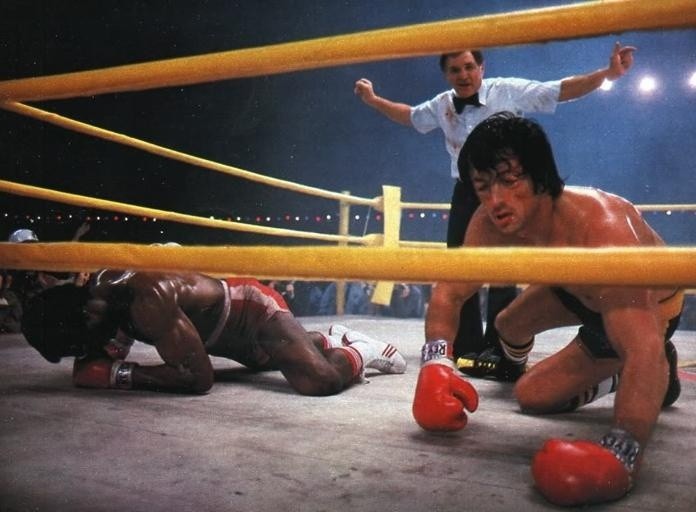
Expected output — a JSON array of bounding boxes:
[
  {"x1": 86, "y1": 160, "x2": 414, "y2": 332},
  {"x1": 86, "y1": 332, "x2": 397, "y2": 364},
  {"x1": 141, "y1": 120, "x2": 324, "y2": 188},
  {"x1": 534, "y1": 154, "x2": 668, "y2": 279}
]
[
  {"x1": 329, "y1": 322, "x2": 406, "y2": 375},
  {"x1": 455, "y1": 353, "x2": 526, "y2": 382},
  {"x1": 663, "y1": 340, "x2": 682, "y2": 411}
]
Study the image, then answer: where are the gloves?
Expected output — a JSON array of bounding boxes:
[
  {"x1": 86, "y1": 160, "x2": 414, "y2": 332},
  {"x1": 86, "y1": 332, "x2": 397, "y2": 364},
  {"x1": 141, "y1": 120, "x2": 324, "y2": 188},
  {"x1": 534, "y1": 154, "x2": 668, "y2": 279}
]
[
  {"x1": 71, "y1": 354, "x2": 115, "y2": 390},
  {"x1": 531, "y1": 437, "x2": 631, "y2": 509},
  {"x1": 412, "y1": 359, "x2": 480, "y2": 432}
]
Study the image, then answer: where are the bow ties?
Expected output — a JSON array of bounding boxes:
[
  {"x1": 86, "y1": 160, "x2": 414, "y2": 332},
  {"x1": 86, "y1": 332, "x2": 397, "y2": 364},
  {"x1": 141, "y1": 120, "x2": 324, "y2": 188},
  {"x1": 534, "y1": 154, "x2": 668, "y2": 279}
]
[{"x1": 451, "y1": 94, "x2": 481, "y2": 115}]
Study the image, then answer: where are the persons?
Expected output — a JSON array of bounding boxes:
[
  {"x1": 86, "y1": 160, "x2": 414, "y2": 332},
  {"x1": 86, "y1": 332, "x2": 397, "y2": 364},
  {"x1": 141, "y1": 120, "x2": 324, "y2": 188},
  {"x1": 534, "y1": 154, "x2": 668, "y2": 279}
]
[
  {"x1": 1, "y1": 226, "x2": 423, "y2": 334},
  {"x1": 22, "y1": 268, "x2": 407, "y2": 397},
  {"x1": 353, "y1": 40, "x2": 641, "y2": 375},
  {"x1": 415, "y1": 109, "x2": 683, "y2": 509}
]
